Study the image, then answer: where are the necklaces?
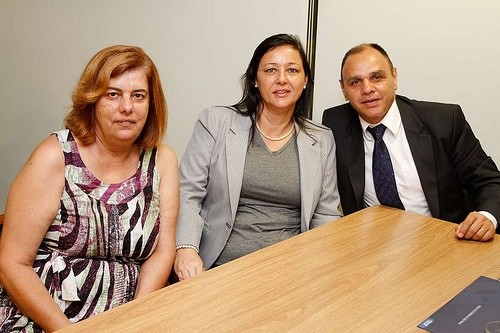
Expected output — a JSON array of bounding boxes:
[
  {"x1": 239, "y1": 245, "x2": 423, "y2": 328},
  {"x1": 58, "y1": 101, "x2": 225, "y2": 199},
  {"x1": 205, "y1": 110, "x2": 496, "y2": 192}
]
[{"x1": 255, "y1": 117, "x2": 296, "y2": 142}]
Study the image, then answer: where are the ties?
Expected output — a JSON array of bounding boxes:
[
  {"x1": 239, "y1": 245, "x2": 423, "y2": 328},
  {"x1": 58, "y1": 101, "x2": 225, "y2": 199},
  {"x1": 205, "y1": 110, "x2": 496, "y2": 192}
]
[{"x1": 366, "y1": 124, "x2": 407, "y2": 211}]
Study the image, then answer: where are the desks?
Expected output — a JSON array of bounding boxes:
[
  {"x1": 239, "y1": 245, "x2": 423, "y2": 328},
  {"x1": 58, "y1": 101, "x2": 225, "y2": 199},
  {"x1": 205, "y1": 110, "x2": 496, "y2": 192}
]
[{"x1": 50, "y1": 205, "x2": 500, "y2": 333}]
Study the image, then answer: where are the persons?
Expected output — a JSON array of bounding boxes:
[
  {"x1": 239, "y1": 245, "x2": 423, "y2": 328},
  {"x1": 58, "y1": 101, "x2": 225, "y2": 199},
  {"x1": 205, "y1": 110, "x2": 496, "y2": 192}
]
[
  {"x1": 321, "y1": 39, "x2": 500, "y2": 241},
  {"x1": 169, "y1": 28, "x2": 346, "y2": 283},
  {"x1": 0, "y1": 43, "x2": 182, "y2": 333}
]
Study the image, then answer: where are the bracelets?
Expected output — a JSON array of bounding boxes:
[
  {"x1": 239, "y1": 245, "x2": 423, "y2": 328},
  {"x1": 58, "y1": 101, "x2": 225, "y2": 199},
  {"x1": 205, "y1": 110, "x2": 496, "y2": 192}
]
[{"x1": 176, "y1": 244, "x2": 198, "y2": 253}]
